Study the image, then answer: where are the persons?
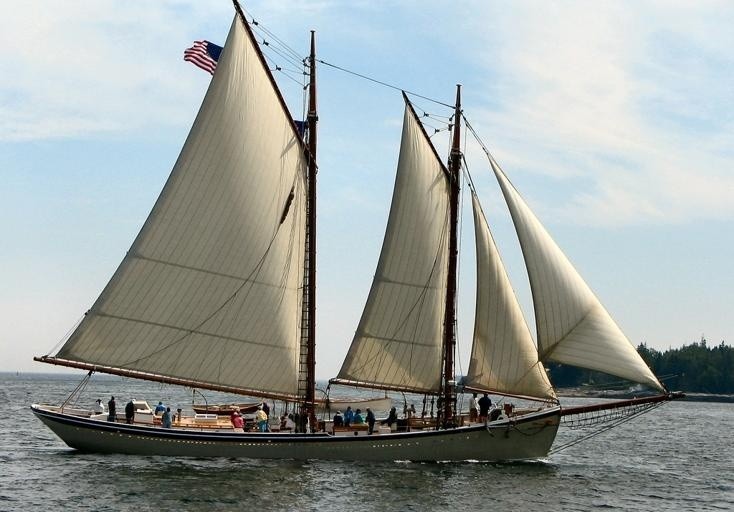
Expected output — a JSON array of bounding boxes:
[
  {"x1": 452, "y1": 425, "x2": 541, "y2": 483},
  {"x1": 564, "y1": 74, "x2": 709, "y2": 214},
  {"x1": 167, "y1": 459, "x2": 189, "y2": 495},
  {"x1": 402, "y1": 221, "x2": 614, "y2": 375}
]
[
  {"x1": 353, "y1": 408, "x2": 364, "y2": 423},
  {"x1": 468, "y1": 392, "x2": 479, "y2": 422},
  {"x1": 125, "y1": 400, "x2": 135, "y2": 424},
  {"x1": 333, "y1": 410, "x2": 343, "y2": 427},
  {"x1": 344, "y1": 406, "x2": 354, "y2": 424},
  {"x1": 162, "y1": 407, "x2": 173, "y2": 429},
  {"x1": 280, "y1": 411, "x2": 320, "y2": 433},
  {"x1": 233, "y1": 401, "x2": 270, "y2": 433},
  {"x1": 381, "y1": 406, "x2": 398, "y2": 433},
  {"x1": 364, "y1": 407, "x2": 376, "y2": 435},
  {"x1": 478, "y1": 392, "x2": 492, "y2": 423},
  {"x1": 108, "y1": 396, "x2": 116, "y2": 422},
  {"x1": 155, "y1": 401, "x2": 167, "y2": 422},
  {"x1": 94, "y1": 398, "x2": 104, "y2": 414}
]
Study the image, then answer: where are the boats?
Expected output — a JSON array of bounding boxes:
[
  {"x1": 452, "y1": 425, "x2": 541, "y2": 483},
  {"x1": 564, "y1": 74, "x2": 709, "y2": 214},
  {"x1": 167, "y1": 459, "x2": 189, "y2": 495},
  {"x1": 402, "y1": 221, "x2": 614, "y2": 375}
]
[
  {"x1": 191, "y1": 403, "x2": 263, "y2": 415},
  {"x1": 299, "y1": 395, "x2": 393, "y2": 413}
]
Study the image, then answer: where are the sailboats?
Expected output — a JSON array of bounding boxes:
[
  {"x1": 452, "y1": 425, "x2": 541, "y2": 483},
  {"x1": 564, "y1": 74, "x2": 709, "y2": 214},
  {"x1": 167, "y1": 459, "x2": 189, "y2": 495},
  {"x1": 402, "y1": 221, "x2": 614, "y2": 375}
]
[{"x1": 27, "y1": 0, "x2": 683, "y2": 463}]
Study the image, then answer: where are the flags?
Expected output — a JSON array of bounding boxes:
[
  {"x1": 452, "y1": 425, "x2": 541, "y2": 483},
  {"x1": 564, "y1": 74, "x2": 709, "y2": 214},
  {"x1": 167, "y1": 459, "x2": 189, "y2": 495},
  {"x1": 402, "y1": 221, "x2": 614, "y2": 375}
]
[{"x1": 182, "y1": 40, "x2": 223, "y2": 77}]
